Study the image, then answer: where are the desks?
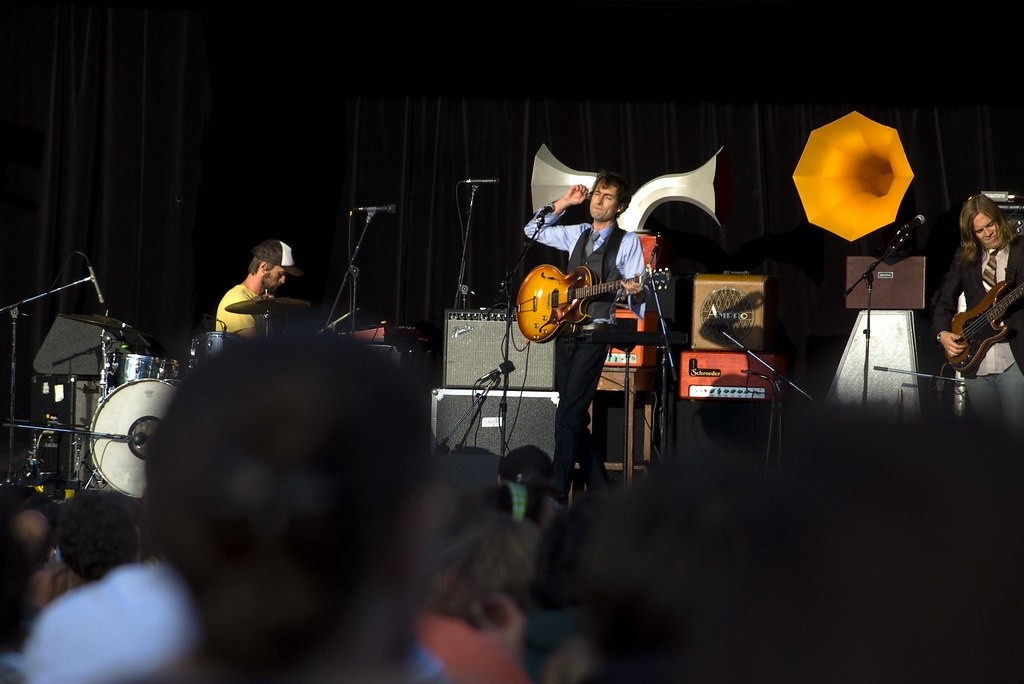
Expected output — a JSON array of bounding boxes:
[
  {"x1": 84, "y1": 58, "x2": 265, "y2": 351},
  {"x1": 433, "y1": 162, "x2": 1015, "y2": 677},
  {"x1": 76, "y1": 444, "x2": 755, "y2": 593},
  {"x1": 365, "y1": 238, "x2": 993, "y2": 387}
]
[{"x1": 568, "y1": 369, "x2": 660, "y2": 506}]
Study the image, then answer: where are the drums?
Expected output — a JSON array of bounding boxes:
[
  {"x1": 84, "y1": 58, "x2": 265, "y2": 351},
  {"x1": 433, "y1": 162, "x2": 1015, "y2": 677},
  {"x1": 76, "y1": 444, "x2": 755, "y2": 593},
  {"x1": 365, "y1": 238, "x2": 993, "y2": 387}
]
[
  {"x1": 117, "y1": 353, "x2": 183, "y2": 388},
  {"x1": 88, "y1": 378, "x2": 178, "y2": 497},
  {"x1": 189, "y1": 331, "x2": 243, "y2": 376}
]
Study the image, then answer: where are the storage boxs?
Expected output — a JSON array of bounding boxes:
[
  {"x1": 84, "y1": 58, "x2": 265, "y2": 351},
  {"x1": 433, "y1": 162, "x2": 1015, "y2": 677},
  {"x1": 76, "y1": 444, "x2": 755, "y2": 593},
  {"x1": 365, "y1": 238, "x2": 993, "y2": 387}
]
[{"x1": 435, "y1": 387, "x2": 562, "y2": 465}]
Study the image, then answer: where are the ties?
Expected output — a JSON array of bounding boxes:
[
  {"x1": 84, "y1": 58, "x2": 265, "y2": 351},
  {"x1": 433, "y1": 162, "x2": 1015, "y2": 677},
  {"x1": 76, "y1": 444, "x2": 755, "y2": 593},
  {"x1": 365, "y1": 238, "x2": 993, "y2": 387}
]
[
  {"x1": 981, "y1": 248, "x2": 999, "y2": 292},
  {"x1": 585, "y1": 232, "x2": 600, "y2": 258}
]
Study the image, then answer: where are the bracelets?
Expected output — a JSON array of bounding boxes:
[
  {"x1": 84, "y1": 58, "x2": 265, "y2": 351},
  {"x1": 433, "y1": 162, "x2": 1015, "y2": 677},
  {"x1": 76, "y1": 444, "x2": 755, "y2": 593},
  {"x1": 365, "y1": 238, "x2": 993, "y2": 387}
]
[{"x1": 937, "y1": 332, "x2": 949, "y2": 344}]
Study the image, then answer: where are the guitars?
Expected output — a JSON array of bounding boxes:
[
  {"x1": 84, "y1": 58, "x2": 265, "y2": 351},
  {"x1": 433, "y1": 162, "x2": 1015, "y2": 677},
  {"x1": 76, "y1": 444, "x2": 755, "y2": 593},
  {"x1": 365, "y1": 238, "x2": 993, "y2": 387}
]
[
  {"x1": 944, "y1": 280, "x2": 1024, "y2": 373},
  {"x1": 516, "y1": 264, "x2": 672, "y2": 344}
]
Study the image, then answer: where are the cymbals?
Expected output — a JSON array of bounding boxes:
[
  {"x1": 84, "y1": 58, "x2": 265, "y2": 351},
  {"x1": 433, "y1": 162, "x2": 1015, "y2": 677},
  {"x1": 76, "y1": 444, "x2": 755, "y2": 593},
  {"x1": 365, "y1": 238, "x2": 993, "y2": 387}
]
[
  {"x1": 58, "y1": 313, "x2": 156, "y2": 338},
  {"x1": 224, "y1": 296, "x2": 311, "y2": 315}
]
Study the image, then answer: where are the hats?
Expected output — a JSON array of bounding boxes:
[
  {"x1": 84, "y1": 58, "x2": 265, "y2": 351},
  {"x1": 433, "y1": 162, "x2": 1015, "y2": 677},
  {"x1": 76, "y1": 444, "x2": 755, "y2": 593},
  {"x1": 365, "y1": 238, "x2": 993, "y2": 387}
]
[
  {"x1": 253, "y1": 240, "x2": 303, "y2": 277},
  {"x1": 501, "y1": 444, "x2": 553, "y2": 486}
]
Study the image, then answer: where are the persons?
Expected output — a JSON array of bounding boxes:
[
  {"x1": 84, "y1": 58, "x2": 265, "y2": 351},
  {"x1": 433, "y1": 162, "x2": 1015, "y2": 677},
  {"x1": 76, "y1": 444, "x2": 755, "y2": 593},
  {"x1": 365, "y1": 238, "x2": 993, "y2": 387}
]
[
  {"x1": 0, "y1": 331, "x2": 1024, "y2": 684},
  {"x1": 215, "y1": 239, "x2": 304, "y2": 342},
  {"x1": 932, "y1": 195, "x2": 1024, "y2": 431},
  {"x1": 522, "y1": 177, "x2": 649, "y2": 514}
]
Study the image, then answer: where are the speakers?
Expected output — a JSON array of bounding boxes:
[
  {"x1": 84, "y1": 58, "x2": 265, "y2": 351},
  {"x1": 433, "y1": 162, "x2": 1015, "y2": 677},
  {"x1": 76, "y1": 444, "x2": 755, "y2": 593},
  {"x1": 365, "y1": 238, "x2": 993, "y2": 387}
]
[
  {"x1": 590, "y1": 391, "x2": 652, "y2": 469},
  {"x1": 33, "y1": 313, "x2": 152, "y2": 376},
  {"x1": 692, "y1": 273, "x2": 765, "y2": 351},
  {"x1": 673, "y1": 400, "x2": 776, "y2": 455},
  {"x1": 443, "y1": 309, "x2": 557, "y2": 392},
  {"x1": 31, "y1": 373, "x2": 104, "y2": 494}
]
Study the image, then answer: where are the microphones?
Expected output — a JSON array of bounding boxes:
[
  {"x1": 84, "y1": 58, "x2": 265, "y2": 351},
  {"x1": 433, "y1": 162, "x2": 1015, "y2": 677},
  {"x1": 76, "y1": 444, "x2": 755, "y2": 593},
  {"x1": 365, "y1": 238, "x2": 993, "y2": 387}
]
[
  {"x1": 86, "y1": 262, "x2": 104, "y2": 303},
  {"x1": 353, "y1": 205, "x2": 397, "y2": 213},
  {"x1": 898, "y1": 214, "x2": 926, "y2": 231},
  {"x1": 203, "y1": 320, "x2": 209, "y2": 329},
  {"x1": 463, "y1": 178, "x2": 497, "y2": 183},
  {"x1": 478, "y1": 361, "x2": 515, "y2": 381},
  {"x1": 537, "y1": 204, "x2": 556, "y2": 220},
  {"x1": 134, "y1": 431, "x2": 146, "y2": 445}
]
